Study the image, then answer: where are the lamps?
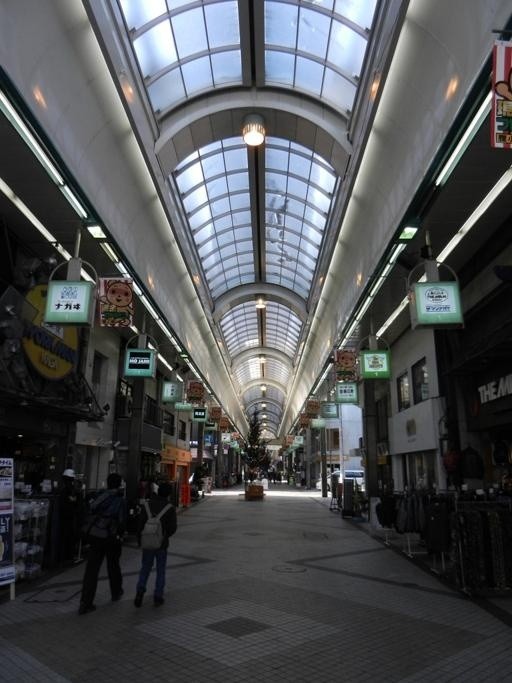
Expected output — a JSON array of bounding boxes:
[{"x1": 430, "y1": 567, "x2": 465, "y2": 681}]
[{"x1": 446, "y1": 445, "x2": 484, "y2": 482}]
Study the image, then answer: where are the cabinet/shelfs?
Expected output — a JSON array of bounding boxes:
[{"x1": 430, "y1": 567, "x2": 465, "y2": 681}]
[{"x1": 16, "y1": 498, "x2": 53, "y2": 593}]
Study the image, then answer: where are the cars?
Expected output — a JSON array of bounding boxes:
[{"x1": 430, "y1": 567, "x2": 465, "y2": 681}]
[{"x1": 316, "y1": 469, "x2": 364, "y2": 490}]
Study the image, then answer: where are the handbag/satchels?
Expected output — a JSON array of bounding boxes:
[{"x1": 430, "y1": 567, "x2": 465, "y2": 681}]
[{"x1": 80, "y1": 515, "x2": 112, "y2": 539}]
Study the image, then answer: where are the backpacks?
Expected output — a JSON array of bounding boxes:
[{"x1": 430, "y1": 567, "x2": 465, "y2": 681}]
[{"x1": 141, "y1": 500, "x2": 174, "y2": 551}]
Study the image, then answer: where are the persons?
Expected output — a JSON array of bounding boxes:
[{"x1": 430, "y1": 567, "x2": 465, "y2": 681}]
[
  {"x1": 131, "y1": 482, "x2": 178, "y2": 609},
  {"x1": 76, "y1": 472, "x2": 126, "y2": 615}
]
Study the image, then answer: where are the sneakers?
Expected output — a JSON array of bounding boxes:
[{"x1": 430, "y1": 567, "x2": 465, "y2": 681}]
[
  {"x1": 79, "y1": 599, "x2": 96, "y2": 614},
  {"x1": 153, "y1": 598, "x2": 164, "y2": 605},
  {"x1": 111, "y1": 589, "x2": 124, "y2": 601},
  {"x1": 134, "y1": 592, "x2": 144, "y2": 608}
]
